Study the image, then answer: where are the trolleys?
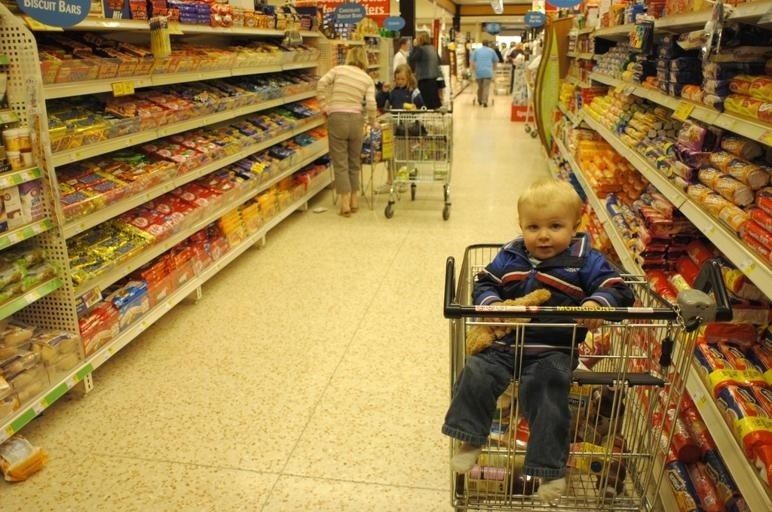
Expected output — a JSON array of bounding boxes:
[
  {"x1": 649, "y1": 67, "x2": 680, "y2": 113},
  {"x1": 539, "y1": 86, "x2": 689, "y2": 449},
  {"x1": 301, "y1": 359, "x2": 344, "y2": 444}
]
[
  {"x1": 444, "y1": 243, "x2": 733, "y2": 510},
  {"x1": 383, "y1": 105, "x2": 454, "y2": 221},
  {"x1": 472, "y1": 63, "x2": 513, "y2": 108}
]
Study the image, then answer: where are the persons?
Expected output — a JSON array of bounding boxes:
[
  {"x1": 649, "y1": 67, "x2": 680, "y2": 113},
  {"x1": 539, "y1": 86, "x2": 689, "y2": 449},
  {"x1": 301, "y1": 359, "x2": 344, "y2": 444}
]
[
  {"x1": 469, "y1": 39, "x2": 499, "y2": 108},
  {"x1": 462, "y1": 38, "x2": 525, "y2": 66},
  {"x1": 381, "y1": 63, "x2": 431, "y2": 183},
  {"x1": 441, "y1": 170, "x2": 636, "y2": 505},
  {"x1": 391, "y1": 37, "x2": 412, "y2": 72},
  {"x1": 315, "y1": 43, "x2": 378, "y2": 215},
  {"x1": 407, "y1": 30, "x2": 442, "y2": 109}
]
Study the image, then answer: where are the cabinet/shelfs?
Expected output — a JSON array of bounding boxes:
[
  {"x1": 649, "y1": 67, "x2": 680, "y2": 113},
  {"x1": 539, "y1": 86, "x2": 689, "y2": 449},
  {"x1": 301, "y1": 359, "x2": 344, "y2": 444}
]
[
  {"x1": 549, "y1": 2, "x2": 770, "y2": 510},
  {"x1": 0, "y1": 2, "x2": 393, "y2": 450}
]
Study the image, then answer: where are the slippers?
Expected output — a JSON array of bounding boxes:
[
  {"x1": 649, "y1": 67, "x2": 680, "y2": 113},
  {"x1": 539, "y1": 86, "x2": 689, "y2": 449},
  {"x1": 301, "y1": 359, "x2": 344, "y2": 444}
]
[{"x1": 338, "y1": 206, "x2": 359, "y2": 217}]
